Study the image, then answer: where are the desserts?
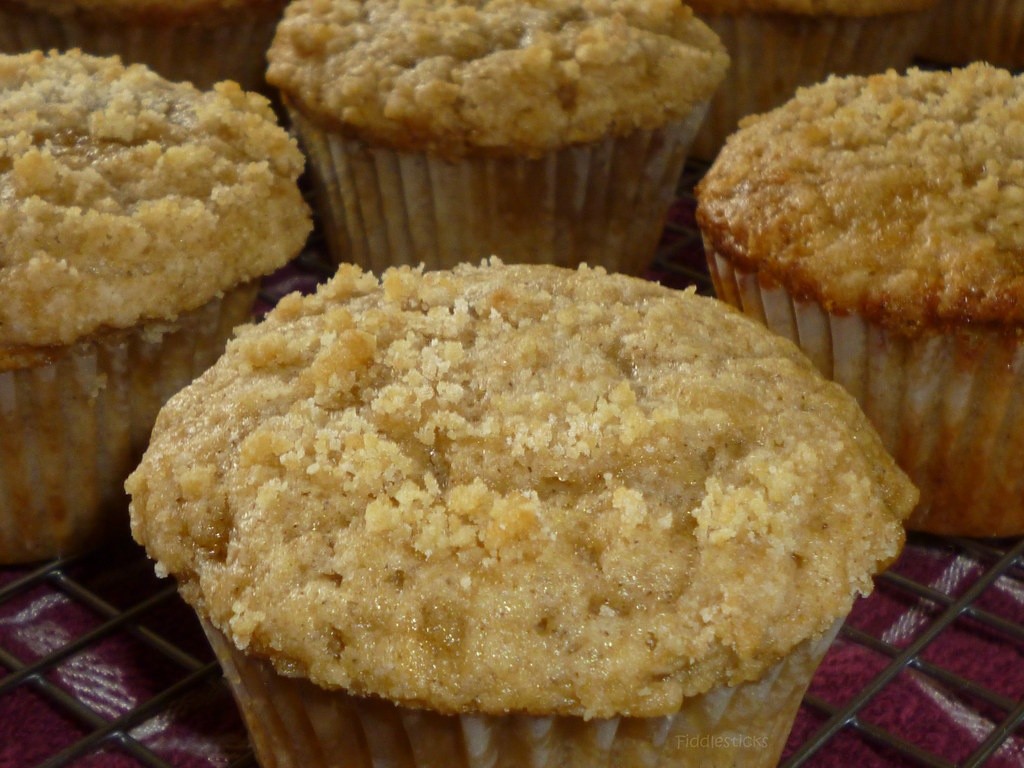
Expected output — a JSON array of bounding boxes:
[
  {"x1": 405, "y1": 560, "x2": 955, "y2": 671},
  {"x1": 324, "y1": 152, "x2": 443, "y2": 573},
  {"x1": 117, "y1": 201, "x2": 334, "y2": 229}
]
[{"x1": 0, "y1": 0, "x2": 1024, "y2": 768}]
